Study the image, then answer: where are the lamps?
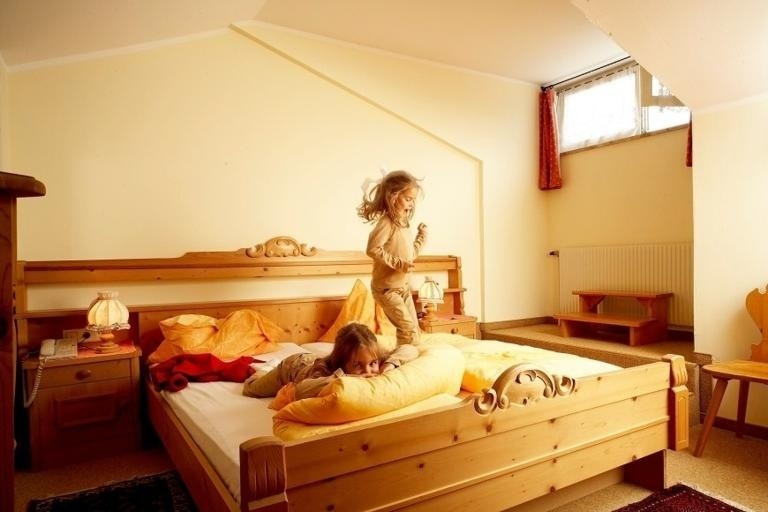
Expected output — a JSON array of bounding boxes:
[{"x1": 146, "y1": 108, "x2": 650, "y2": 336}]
[
  {"x1": 85, "y1": 289, "x2": 133, "y2": 352},
  {"x1": 415, "y1": 277, "x2": 445, "y2": 322}
]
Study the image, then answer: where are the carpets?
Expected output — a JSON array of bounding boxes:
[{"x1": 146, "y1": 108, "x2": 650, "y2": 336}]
[
  {"x1": 20, "y1": 469, "x2": 197, "y2": 510},
  {"x1": 611, "y1": 481, "x2": 761, "y2": 512}
]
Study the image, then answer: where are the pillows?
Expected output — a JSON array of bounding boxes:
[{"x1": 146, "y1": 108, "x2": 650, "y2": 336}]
[
  {"x1": 146, "y1": 307, "x2": 283, "y2": 362},
  {"x1": 318, "y1": 280, "x2": 399, "y2": 342}
]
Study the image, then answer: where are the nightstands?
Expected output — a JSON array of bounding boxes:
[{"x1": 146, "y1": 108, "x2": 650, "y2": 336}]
[
  {"x1": 418, "y1": 313, "x2": 479, "y2": 340},
  {"x1": 20, "y1": 341, "x2": 143, "y2": 472}
]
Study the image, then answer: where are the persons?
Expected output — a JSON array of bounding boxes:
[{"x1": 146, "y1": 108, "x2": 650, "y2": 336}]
[
  {"x1": 242, "y1": 322, "x2": 419, "y2": 398},
  {"x1": 356, "y1": 170, "x2": 428, "y2": 349}
]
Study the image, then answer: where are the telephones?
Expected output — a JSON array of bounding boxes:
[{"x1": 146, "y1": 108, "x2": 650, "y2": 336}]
[{"x1": 38, "y1": 339, "x2": 78, "y2": 361}]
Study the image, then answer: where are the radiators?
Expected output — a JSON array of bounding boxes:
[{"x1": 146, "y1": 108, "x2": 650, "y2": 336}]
[{"x1": 555, "y1": 242, "x2": 693, "y2": 330}]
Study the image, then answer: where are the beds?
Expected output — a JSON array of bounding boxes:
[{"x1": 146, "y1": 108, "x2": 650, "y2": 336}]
[{"x1": 137, "y1": 297, "x2": 678, "y2": 511}]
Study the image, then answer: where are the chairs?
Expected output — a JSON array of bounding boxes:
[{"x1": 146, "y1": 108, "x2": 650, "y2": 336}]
[{"x1": 694, "y1": 282, "x2": 768, "y2": 459}]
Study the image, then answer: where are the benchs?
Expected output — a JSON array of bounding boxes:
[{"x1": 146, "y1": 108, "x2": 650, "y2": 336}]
[{"x1": 550, "y1": 288, "x2": 674, "y2": 346}]
